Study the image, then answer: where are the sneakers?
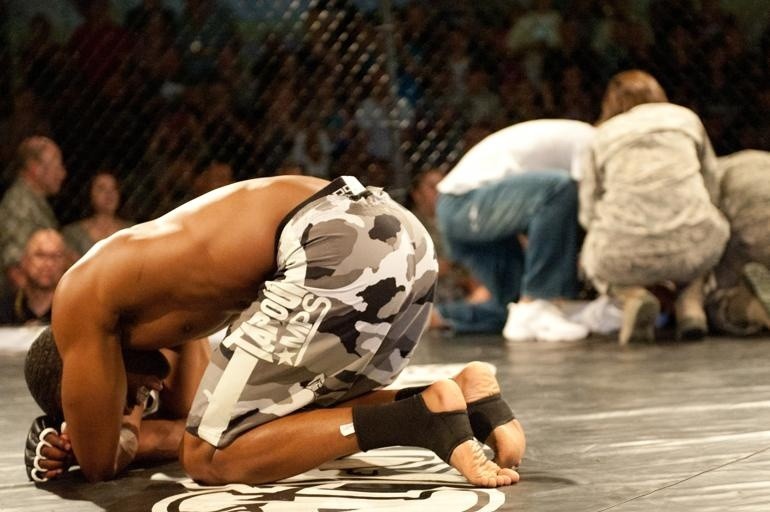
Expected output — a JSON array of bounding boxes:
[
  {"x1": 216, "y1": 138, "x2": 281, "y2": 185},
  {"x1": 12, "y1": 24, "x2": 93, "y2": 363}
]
[
  {"x1": 672, "y1": 300, "x2": 707, "y2": 339},
  {"x1": 734, "y1": 263, "x2": 770, "y2": 333},
  {"x1": 618, "y1": 290, "x2": 659, "y2": 344},
  {"x1": 503, "y1": 300, "x2": 589, "y2": 341}
]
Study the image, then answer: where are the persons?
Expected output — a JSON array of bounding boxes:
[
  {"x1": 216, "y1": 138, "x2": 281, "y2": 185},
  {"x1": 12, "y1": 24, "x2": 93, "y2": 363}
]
[
  {"x1": 0, "y1": 228, "x2": 69, "y2": 325},
  {"x1": 574, "y1": 69, "x2": 729, "y2": 344},
  {"x1": 3, "y1": 1, "x2": 770, "y2": 189},
  {"x1": 1, "y1": 134, "x2": 70, "y2": 241},
  {"x1": 429, "y1": 116, "x2": 598, "y2": 345},
  {"x1": 25, "y1": 172, "x2": 526, "y2": 487},
  {"x1": 705, "y1": 148, "x2": 770, "y2": 339},
  {"x1": 61, "y1": 171, "x2": 139, "y2": 269}
]
